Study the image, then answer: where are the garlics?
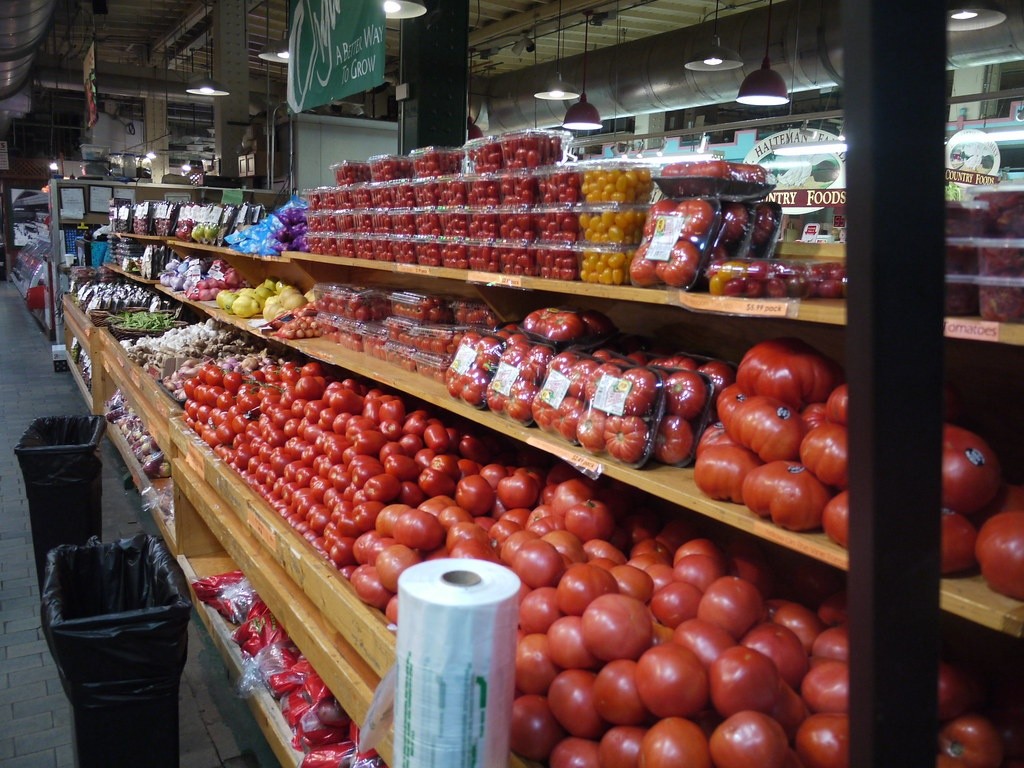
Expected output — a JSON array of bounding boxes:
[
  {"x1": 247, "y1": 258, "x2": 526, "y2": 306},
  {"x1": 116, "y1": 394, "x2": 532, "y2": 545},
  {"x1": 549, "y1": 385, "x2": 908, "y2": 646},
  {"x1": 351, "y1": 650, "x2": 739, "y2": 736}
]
[
  {"x1": 161, "y1": 257, "x2": 209, "y2": 291},
  {"x1": 117, "y1": 317, "x2": 234, "y2": 351}
]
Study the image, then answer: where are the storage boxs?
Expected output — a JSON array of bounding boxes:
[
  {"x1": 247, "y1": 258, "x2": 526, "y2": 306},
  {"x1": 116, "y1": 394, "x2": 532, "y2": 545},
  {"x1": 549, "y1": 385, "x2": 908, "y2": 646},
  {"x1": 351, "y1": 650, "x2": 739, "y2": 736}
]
[
  {"x1": 106, "y1": 153, "x2": 136, "y2": 179},
  {"x1": 247, "y1": 154, "x2": 281, "y2": 177},
  {"x1": 79, "y1": 144, "x2": 112, "y2": 160},
  {"x1": 51, "y1": 345, "x2": 67, "y2": 372},
  {"x1": 79, "y1": 161, "x2": 109, "y2": 175},
  {"x1": 237, "y1": 155, "x2": 246, "y2": 177},
  {"x1": 162, "y1": 176, "x2": 190, "y2": 184}
]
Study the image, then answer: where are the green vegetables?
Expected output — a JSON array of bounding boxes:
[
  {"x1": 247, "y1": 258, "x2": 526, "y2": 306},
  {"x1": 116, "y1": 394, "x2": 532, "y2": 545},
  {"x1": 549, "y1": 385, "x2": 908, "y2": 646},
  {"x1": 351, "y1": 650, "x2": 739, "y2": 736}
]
[{"x1": 105, "y1": 312, "x2": 176, "y2": 330}]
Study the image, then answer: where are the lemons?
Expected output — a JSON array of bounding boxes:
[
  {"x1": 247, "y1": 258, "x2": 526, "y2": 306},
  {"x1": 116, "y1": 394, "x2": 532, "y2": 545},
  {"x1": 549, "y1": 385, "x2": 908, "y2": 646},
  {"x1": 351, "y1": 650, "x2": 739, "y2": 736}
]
[{"x1": 217, "y1": 274, "x2": 293, "y2": 318}]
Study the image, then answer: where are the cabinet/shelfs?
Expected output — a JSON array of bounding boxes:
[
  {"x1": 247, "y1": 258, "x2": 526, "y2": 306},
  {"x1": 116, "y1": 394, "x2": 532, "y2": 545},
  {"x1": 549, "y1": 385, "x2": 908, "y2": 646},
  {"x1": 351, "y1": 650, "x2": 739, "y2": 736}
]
[{"x1": 64, "y1": 232, "x2": 1024, "y2": 768}]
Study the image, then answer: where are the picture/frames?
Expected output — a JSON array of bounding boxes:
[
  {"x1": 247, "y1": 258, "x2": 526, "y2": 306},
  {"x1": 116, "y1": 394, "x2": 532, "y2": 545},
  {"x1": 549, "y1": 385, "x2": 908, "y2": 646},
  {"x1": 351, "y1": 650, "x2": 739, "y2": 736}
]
[
  {"x1": 165, "y1": 194, "x2": 191, "y2": 205},
  {"x1": 89, "y1": 185, "x2": 118, "y2": 216},
  {"x1": 60, "y1": 187, "x2": 86, "y2": 216}
]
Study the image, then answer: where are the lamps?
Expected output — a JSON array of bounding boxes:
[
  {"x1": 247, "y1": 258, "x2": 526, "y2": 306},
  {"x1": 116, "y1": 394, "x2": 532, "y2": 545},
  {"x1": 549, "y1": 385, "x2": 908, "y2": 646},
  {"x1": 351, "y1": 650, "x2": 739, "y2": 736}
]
[
  {"x1": 184, "y1": 0, "x2": 230, "y2": 97},
  {"x1": 735, "y1": 1, "x2": 789, "y2": 106},
  {"x1": 258, "y1": 0, "x2": 292, "y2": 64},
  {"x1": 563, "y1": 11, "x2": 604, "y2": 131},
  {"x1": 383, "y1": 0, "x2": 427, "y2": 19},
  {"x1": 946, "y1": 1, "x2": 1008, "y2": 32},
  {"x1": 534, "y1": 13, "x2": 580, "y2": 101}
]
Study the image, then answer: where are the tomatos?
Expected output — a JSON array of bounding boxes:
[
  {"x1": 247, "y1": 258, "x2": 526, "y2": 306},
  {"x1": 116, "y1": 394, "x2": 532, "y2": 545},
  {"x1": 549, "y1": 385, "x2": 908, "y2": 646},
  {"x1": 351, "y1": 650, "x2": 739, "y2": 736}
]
[
  {"x1": 303, "y1": 125, "x2": 1024, "y2": 322},
  {"x1": 178, "y1": 287, "x2": 1024, "y2": 768}
]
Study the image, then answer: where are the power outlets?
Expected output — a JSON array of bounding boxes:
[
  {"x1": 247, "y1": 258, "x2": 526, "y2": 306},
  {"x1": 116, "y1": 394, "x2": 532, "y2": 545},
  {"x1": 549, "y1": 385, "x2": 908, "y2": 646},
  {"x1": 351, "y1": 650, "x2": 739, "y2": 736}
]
[{"x1": 684, "y1": 0, "x2": 741, "y2": 71}]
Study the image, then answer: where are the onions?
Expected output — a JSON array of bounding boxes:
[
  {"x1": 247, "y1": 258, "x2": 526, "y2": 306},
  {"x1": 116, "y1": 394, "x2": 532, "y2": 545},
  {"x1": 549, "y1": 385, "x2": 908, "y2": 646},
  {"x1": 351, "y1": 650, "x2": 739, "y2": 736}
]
[{"x1": 161, "y1": 347, "x2": 291, "y2": 401}]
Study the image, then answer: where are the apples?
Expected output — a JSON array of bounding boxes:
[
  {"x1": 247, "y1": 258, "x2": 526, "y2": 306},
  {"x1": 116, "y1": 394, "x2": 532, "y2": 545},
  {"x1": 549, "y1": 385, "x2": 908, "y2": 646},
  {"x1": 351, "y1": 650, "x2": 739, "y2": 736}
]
[{"x1": 105, "y1": 395, "x2": 171, "y2": 478}]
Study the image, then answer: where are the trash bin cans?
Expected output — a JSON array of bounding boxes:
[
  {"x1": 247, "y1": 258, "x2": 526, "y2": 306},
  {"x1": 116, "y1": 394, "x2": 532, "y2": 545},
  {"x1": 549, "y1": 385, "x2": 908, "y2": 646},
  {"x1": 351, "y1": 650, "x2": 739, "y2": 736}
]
[
  {"x1": 12, "y1": 414, "x2": 110, "y2": 551},
  {"x1": 36, "y1": 535, "x2": 195, "y2": 768}
]
